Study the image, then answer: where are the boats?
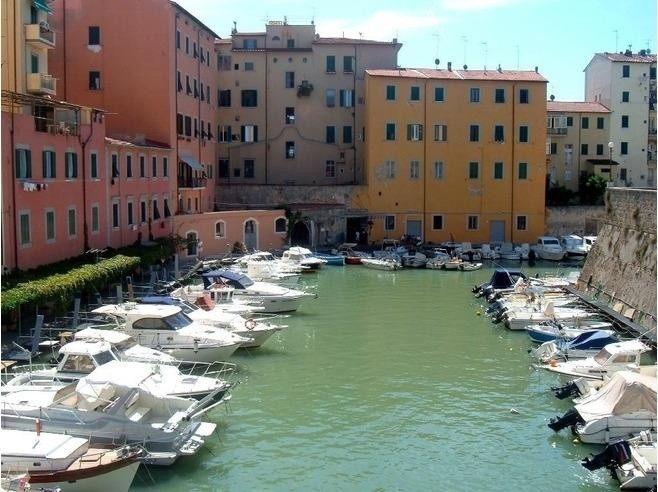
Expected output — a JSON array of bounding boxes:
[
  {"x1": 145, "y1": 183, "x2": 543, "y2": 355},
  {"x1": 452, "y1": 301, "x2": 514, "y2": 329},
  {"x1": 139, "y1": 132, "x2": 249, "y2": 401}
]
[{"x1": 346, "y1": 234, "x2": 597, "y2": 270}]
